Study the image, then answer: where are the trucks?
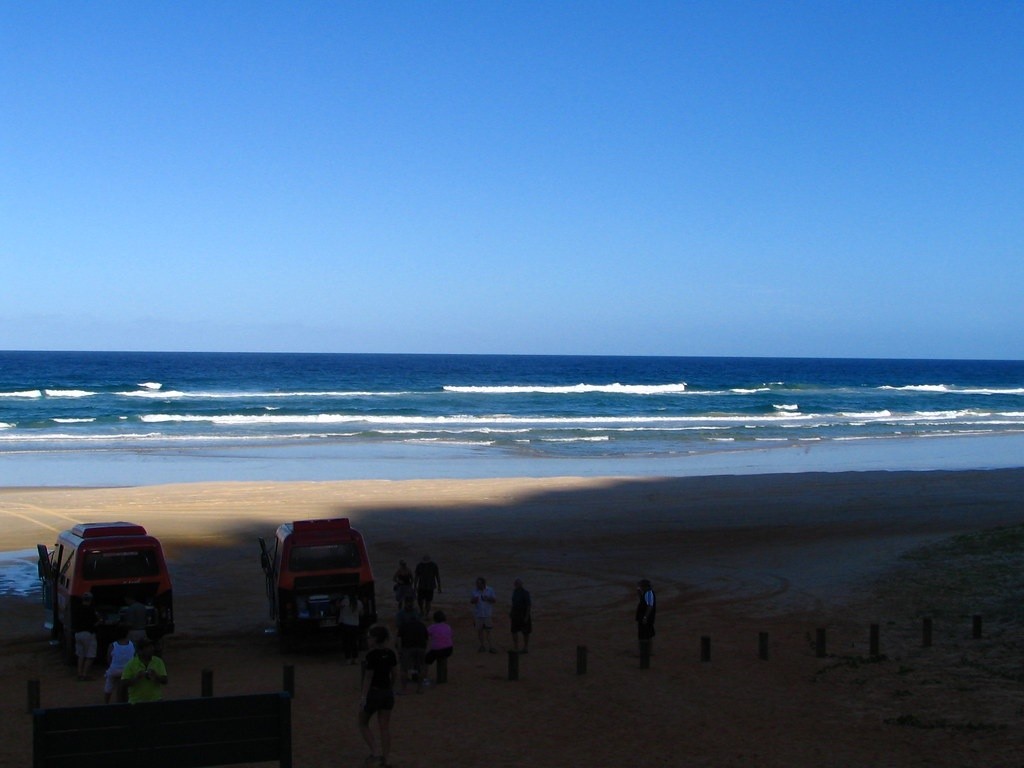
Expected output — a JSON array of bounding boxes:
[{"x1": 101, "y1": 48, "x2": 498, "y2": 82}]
[
  {"x1": 259, "y1": 517, "x2": 377, "y2": 642},
  {"x1": 37, "y1": 521, "x2": 175, "y2": 652}
]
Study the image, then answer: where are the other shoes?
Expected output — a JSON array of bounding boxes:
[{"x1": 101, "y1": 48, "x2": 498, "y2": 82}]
[
  {"x1": 521, "y1": 648, "x2": 528, "y2": 654},
  {"x1": 478, "y1": 646, "x2": 487, "y2": 652},
  {"x1": 78, "y1": 675, "x2": 91, "y2": 681},
  {"x1": 489, "y1": 648, "x2": 496, "y2": 653},
  {"x1": 416, "y1": 689, "x2": 423, "y2": 694},
  {"x1": 375, "y1": 764, "x2": 391, "y2": 768},
  {"x1": 424, "y1": 616, "x2": 429, "y2": 620},
  {"x1": 365, "y1": 755, "x2": 385, "y2": 763},
  {"x1": 395, "y1": 691, "x2": 408, "y2": 695}
]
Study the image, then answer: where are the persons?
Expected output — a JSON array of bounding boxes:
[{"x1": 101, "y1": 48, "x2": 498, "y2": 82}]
[
  {"x1": 102, "y1": 593, "x2": 169, "y2": 704},
  {"x1": 359, "y1": 625, "x2": 398, "y2": 768},
  {"x1": 389, "y1": 551, "x2": 454, "y2": 695},
  {"x1": 469, "y1": 577, "x2": 498, "y2": 653},
  {"x1": 74, "y1": 592, "x2": 103, "y2": 679},
  {"x1": 506, "y1": 577, "x2": 532, "y2": 653},
  {"x1": 628, "y1": 579, "x2": 656, "y2": 657}
]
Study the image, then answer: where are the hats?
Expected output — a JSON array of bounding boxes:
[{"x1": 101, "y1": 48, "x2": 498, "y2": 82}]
[
  {"x1": 134, "y1": 630, "x2": 153, "y2": 645},
  {"x1": 82, "y1": 592, "x2": 93, "y2": 597},
  {"x1": 421, "y1": 555, "x2": 433, "y2": 563}
]
